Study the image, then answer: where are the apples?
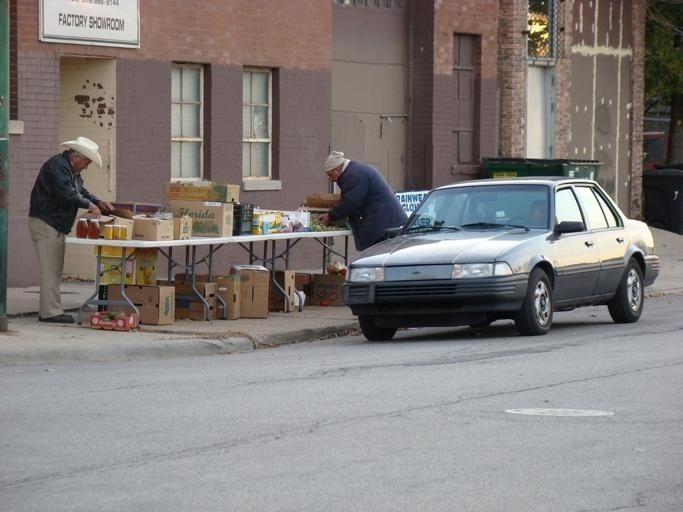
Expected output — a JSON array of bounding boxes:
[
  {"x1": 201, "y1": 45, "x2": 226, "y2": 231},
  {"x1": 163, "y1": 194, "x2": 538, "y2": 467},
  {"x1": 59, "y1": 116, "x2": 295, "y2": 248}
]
[
  {"x1": 328, "y1": 261, "x2": 347, "y2": 274},
  {"x1": 321, "y1": 293, "x2": 338, "y2": 306}
]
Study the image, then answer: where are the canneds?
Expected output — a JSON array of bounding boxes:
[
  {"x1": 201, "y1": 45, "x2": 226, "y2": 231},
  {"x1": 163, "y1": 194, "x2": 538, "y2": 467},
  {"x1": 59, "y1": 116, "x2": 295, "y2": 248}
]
[
  {"x1": 76, "y1": 219, "x2": 100, "y2": 238},
  {"x1": 105, "y1": 224, "x2": 127, "y2": 240}
]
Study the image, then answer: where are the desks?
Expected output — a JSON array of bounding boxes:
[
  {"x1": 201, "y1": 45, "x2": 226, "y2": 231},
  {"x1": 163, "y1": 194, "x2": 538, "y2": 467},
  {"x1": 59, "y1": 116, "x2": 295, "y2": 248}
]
[{"x1": 64, "y1": 226, "x2": 354, "y2": 323}]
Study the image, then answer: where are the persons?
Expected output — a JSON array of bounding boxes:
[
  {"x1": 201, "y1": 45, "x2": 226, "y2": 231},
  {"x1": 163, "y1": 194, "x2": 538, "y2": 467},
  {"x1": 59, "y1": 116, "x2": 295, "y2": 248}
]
[
  {"x1": 528, "y1": 200, "x2": 549, "y2": 226},
  {"x1": 27, "y1": 137, "x2": 115, "y2": 323},
  {"x1": 315, "y1": 149, "x2": 411, "y2": 254}
]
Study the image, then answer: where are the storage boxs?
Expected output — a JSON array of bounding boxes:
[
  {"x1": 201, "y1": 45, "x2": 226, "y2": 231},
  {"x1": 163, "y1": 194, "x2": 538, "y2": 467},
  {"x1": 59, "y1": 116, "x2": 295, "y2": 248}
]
[{"x1": 99, "y1": 182, "x2": 346, "y2": 327}]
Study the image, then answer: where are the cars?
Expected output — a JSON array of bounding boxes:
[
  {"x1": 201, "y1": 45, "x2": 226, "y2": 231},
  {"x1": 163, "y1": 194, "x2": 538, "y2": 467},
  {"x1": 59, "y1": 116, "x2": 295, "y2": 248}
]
[{"x1": 343, "y1": 177, "x2": 661, "y2": 338}]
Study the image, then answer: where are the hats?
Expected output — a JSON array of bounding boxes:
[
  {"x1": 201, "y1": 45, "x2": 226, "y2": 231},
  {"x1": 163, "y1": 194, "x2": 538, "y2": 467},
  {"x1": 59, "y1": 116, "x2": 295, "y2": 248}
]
[
  {"x1": 61, "y1": 137, "x2": 102, "y2": 168},
  {"x1": 325, "y1": 151, "x2": 345, "y2": 172}
]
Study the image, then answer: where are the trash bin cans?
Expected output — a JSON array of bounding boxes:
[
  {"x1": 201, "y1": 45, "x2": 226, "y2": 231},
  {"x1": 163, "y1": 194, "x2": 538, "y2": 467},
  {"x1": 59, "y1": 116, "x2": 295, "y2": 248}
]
[
  {"x1": 481, "y1": 157, "x2": 526, "y2": 178},
  {"x1": 527, "y1": 159, "x2": 605, "y2": 186}
]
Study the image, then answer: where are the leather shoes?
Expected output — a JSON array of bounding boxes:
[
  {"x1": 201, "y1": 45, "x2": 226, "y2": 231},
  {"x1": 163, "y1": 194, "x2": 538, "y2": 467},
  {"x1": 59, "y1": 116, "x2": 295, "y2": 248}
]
[{"x1": 39, "y1": 314, "x2": 73, "y2": 323}]
